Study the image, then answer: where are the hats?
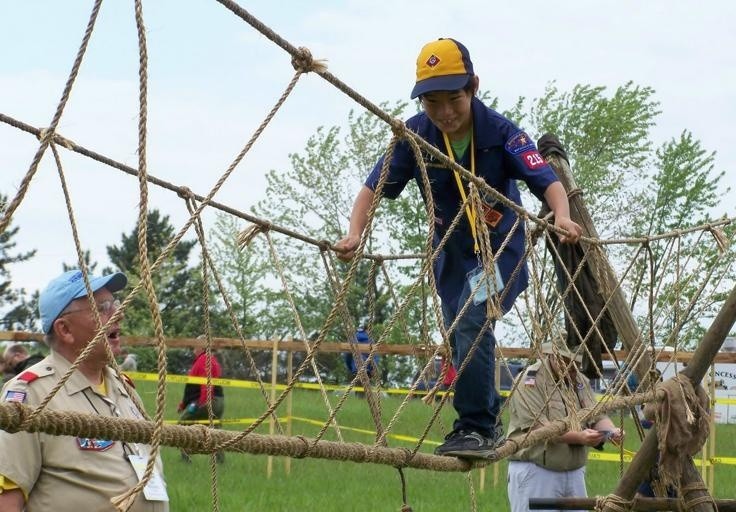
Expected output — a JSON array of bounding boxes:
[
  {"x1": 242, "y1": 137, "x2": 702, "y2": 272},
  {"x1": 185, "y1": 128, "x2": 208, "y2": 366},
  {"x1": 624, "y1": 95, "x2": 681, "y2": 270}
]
[
  {"x1": 538, "y1": 330, "x2": 586, "y2": 363},
  {"x1": 410, "y1": 37, "x2": 473, "y2": 99},
  {"x1": 38, "y1": 270, "x2": 126, "y2": 335},
  {"x1": 357, "y1": 321, "x2": 368, "y2": 332}
]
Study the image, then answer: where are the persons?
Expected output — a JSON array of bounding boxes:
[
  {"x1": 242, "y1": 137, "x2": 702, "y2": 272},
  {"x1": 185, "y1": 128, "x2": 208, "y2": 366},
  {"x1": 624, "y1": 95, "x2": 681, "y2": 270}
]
[
  {"x1": 630, "y1": 368, "x2": 683, "y2": 498},
  {"x1": 338, "y1": 37, "x2": 582, "y2": 458},
  {"x1": 345, "y1": 317, "x2": 379, "y2": 399},
  {"x1": 176, "y1": 335, "x2": 225, "y2": 464},
  {"x1": 120, "y1": 350, "x2": 138, "y2": 371},
  {"x1": 442, "y1": 358, "x2": 457, "y2": 402},
  {"x1": 0, "y1": 271, "x2": 170, "y2": 512},
  {"x1": 505, "y1": 328, "x2": 626, "y2": 512},
  {"x1": 3, "y1": 345, "x2": 45, "y2": 374}
]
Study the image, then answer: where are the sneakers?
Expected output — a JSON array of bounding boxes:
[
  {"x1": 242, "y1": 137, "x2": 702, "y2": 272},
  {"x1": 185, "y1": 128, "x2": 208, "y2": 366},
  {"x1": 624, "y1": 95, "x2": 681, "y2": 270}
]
[
  {"x1": 433, "y1": 426, "x2": 465, "y2": 456},
  {"x1": 438, "y1": 421, "x2": 506, "y2": 460}
]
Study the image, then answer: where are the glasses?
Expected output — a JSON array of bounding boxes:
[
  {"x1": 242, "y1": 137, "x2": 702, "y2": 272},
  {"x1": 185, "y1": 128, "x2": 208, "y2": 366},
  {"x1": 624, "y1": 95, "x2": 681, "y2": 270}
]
[{"x1": 59, "y1": 299, "x2": 120, "y2": 318}]
